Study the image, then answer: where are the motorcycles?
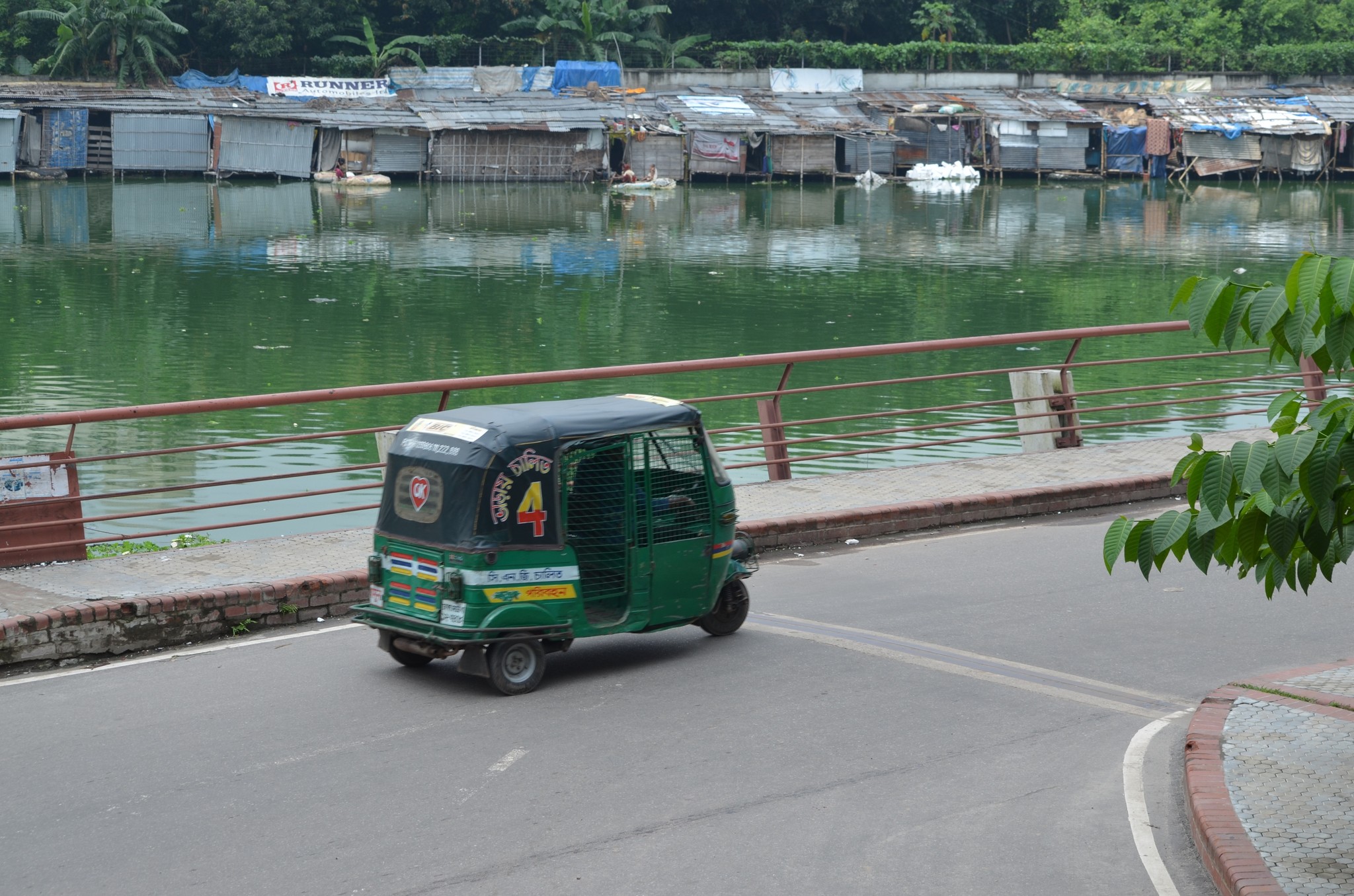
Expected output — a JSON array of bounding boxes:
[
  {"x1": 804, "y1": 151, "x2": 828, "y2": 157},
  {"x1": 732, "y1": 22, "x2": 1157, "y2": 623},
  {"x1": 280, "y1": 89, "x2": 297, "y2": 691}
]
[{"x1": 347, "y1": 392, "x2": 760, "y2": 698}]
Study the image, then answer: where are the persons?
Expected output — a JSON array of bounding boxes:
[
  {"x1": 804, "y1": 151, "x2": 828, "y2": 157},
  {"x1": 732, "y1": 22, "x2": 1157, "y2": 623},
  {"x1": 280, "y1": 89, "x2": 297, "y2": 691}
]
[
  {"x1": 612, "y1": 164, "x2": 637, "y2": 183},
  {"x1": 635, "y1": 480, "x2": 697, "y2": 510},
  {"x1": 335, "y1": 158, "x2": 347, "y2": 181},
  {"x1": 639, "y1": 164, "x2": 655, "y2": 181}
]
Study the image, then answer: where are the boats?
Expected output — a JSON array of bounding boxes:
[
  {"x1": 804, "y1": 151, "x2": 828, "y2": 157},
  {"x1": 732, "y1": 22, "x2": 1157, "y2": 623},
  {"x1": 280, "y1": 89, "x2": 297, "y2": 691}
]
[
  {"x1": 613, "y1": 177, "x2": 677, "y2": 189},
  {"x1": 313, "y1": 171, "x2": 392, "y2": 187}
]
